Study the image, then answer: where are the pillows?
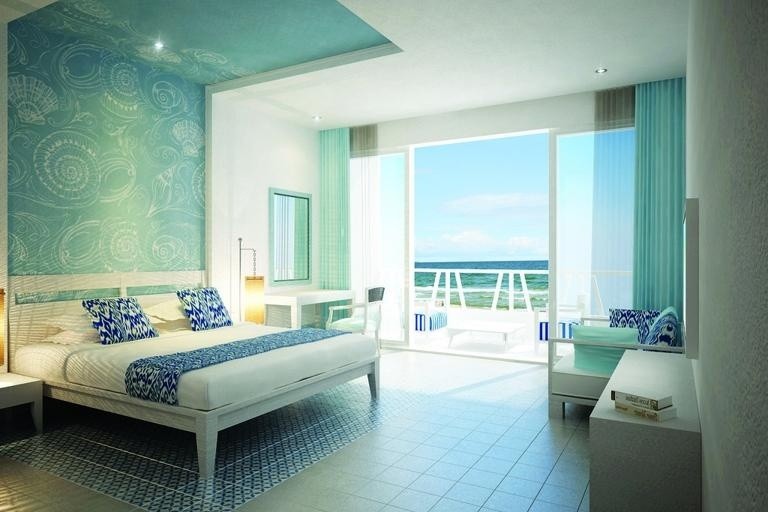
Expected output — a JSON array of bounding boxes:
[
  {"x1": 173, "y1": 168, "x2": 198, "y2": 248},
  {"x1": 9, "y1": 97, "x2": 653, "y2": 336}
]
[{"x1": 82, "y1": 287, "x2": 232, "y2": 346}]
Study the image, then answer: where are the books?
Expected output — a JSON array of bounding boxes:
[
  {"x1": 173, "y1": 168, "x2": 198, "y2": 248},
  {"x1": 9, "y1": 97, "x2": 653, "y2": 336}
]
[{"x1": 611, "y1": 386, "x2": 679, "y2": 422}]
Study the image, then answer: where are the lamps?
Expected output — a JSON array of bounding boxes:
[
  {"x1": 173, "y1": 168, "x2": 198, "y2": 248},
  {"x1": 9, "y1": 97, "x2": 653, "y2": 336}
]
[{"x1": 238, "y1": 236, "x2": 265, "y2": 325}]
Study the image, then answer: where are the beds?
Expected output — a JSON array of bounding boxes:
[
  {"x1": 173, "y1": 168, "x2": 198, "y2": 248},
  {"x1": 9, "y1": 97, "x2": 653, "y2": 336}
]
[{"x1": 13, "y1": 321, "x2": 380, "y2": 479}]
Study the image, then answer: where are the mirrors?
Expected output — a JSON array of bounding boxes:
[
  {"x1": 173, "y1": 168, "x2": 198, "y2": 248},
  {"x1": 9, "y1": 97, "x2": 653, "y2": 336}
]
[{"x1": 266, "y1": 185, "x2": 314, "y2": 287}]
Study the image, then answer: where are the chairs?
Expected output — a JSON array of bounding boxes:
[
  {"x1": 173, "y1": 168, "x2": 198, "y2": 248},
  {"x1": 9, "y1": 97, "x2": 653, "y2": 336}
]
[
  {"x1": 535, "y1": 294, "x2": 685, "y2": 419},
  {"x1": 326, "y1": 287, "x2": 385, "y2": 358},
  {"x1": 414, "y1": 298, "x2": 447, "y2": 331}
]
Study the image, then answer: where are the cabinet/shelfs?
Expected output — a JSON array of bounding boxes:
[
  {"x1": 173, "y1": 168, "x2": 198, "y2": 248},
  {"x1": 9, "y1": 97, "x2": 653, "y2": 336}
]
[{"x1": 589, "y1": 348, "x2": 702, "y2": 512}]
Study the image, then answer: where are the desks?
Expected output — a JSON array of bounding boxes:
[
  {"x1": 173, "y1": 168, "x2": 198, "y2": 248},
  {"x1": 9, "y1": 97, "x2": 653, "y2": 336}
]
[
  {"x1": 0, "y1": 372, "x2": 43, "y2": 437},
  {"x1": 444, "y1": 321, "x2": 528, "y2": 350},
  {"x1": 263, "y1": 288, "x2": 354, "y2": 331}
]
